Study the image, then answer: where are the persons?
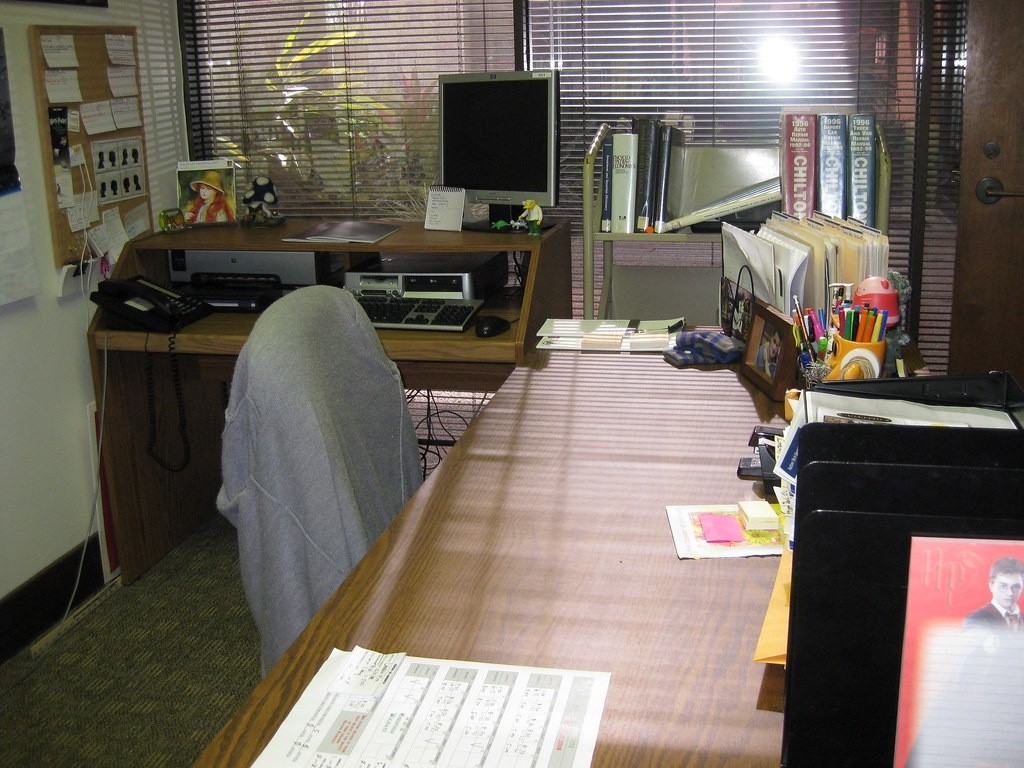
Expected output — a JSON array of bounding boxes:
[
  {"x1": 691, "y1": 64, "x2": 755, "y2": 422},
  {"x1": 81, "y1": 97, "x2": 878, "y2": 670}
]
[
  {"x1": 966, "y1": 556, "x2": 1024, "y2": 638},
  {"x1": 755, "y1": 330, "x2": 783, "y2": 379},
  {"x1": 731, "y1": 292, "x2": 746, "y2": 338}
]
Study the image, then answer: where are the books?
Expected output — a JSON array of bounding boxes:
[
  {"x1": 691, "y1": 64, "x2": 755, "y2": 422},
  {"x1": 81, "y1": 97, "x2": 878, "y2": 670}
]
[{"x1": 281, "y1": 220, "x2": 404, "y2": 244}]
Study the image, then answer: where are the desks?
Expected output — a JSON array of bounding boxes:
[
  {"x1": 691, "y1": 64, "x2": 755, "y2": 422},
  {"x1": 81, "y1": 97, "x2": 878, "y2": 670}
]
[
  {"x1": 86, "y1": 220, "x2": 572, "y2": 586},
  {"x1": 192, "y1": 319, "x2": 791, "y2": 768}
]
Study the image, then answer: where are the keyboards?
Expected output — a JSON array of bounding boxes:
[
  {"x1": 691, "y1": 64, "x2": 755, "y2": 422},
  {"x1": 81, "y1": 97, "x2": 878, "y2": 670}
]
[{"x1": 356, "y1": 295, "x2": 484, "y2": 332}]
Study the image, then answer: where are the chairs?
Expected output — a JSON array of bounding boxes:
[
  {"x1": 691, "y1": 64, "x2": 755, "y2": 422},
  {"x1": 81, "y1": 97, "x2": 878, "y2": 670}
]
[{"x1": 215, "y1": 286, "x2": 421, "y2": 684}]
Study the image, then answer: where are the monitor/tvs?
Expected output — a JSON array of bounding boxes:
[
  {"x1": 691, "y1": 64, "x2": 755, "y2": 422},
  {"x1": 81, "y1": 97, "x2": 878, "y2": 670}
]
[{"x1": 440, "y1": 69, "x2": 561, "y2": 233}]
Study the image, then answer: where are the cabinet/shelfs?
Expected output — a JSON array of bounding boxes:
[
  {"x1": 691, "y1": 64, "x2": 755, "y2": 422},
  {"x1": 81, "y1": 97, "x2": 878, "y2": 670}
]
[{"x1": 582, "y1": 113, "x2": 892, "y2": 320}]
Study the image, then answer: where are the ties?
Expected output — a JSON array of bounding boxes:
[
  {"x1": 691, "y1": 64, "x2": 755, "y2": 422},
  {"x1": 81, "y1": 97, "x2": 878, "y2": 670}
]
[{"x1": 1006, "y1": 613, "x2": 1016, "y2": 627}]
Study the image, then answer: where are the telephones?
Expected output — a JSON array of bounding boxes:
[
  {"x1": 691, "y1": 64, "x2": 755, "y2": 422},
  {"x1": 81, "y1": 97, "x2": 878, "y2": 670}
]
[{"x1": 90, "y1": 274, "x2": 217, "y2": 333}]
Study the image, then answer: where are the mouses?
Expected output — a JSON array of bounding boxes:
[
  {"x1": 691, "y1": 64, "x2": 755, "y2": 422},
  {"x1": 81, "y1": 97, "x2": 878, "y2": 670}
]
[{"x1": 475, "y1": 315, "x2": 511, "y2": 338}]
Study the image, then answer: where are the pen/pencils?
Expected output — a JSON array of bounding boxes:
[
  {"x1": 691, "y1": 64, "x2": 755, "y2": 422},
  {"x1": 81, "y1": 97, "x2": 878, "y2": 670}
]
[{"x1": 787, "y1": 281, "x2": 891, "y2": 387}]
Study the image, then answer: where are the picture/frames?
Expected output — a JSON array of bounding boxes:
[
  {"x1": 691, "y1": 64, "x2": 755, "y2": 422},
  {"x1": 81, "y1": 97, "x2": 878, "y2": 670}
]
[{"x1": 740, "y1": 304, "x2": 798, "y2": 404}]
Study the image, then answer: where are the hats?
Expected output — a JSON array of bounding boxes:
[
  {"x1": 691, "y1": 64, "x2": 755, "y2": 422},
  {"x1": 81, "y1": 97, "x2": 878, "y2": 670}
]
[{"x1": 190, "y1": 172, "x2": 226, "y2": 197}]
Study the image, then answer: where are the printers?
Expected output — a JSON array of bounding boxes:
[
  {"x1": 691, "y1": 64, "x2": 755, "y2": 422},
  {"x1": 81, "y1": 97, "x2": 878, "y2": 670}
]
[{"x1": 168, "y1": 249, "x2": 342, "y2": 312}]
